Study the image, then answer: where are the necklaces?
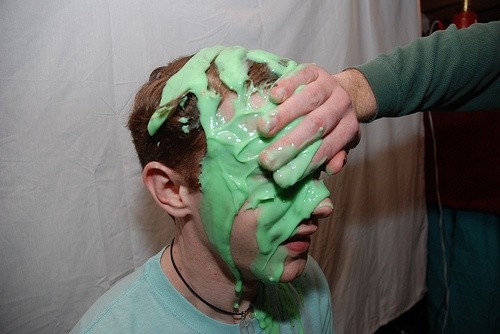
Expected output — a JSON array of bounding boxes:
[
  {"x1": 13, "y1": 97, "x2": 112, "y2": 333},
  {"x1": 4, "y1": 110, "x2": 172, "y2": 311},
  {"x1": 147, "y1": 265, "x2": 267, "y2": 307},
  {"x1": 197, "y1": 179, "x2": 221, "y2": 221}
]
[{"x1": 169, "y1": 236, "x2": 264, "y2": 334}]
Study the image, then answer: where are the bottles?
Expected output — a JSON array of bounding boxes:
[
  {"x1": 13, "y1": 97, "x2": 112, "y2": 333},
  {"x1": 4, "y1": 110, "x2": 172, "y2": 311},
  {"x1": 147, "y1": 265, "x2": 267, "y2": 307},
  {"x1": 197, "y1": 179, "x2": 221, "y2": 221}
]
[{"x1": 452, "y1": 0, "x2": 477, "y2": 29}]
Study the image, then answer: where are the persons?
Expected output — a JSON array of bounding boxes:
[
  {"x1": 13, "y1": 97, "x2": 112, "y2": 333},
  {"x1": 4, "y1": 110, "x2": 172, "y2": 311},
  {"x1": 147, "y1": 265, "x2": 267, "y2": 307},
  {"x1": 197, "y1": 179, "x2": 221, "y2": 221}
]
[
  {"x1": 69, "y1": 46, "x2": 334, "y2": 334},
  {"x1": 256, "y1": 21, "x2": 500, "y2": 188},
  {"x1": 423, "y1": 111, "x2": 500, "y2": 334}
]
